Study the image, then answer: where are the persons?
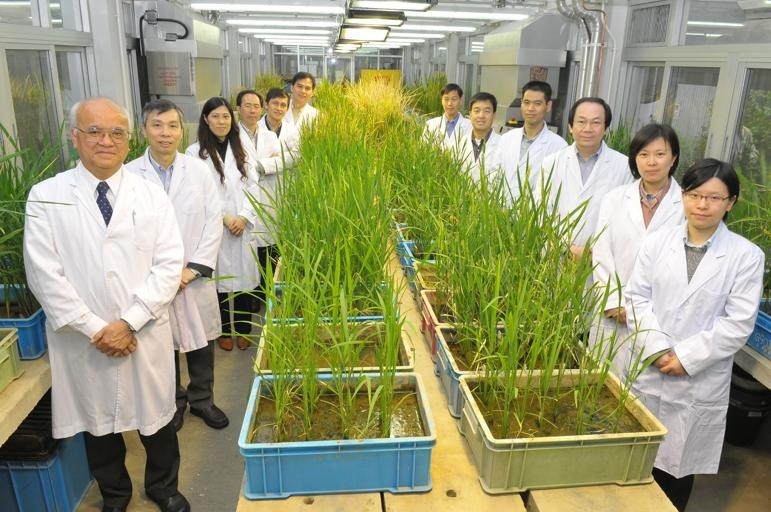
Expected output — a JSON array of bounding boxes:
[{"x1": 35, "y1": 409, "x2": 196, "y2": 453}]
[
  {"x1": 236, "y1": 90, "x2": 299, "y2": 314},
  {"x1": 420, "y1": 83, "x2": 471, "y2": 152},
  {"x1": 282, "y1": 71, "x2": 320, "y2": 136},
  {"x1": 589, "y1": 123, "x2": 686, "y2": 390},
  {"x1": 257, "y1": 87, "x2": 300, "y2": 168},
  {"x1": 123, "y1": 99, "x2": 229, "y2": 432},
  {"x1": 491, "y1": 81, "x2": 569, "y2": 205},
  {"x1": 185, "y1": 96, "x2": 261, "y2": 351},
  {"x1": 539, "y1": 97, "x2": 634, "y2": 344},
  {"x1": 23, "y1": 96, "x2": 190, "y2": 512},
  {"x1": 612, "y1": 157, "x2": 765, "y2": 512},
  {"x1": 457, "y1": 92, "x2": 501, "y2": 187}
]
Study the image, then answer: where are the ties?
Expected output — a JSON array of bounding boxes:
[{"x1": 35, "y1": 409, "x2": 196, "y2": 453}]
[{"x1": 96, "y1": 183, "x2": 113, "y2": 228}]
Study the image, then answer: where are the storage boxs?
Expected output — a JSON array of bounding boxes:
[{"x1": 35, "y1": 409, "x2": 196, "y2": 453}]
[
  {"x1": 0, "y1": 402, "x2": 96, "y2": 511},
  {"x1": 0, "y1": 328, "x2": 28, "y2": 397}
]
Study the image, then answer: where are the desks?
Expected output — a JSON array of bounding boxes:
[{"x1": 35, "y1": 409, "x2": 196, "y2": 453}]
[
  {"x1": 732, "y1": 343, "x2": 771, "y2": 392},
  {"x1": 234, "y1": 229, "x2": 680, "y2": 512},
  {"x1": 1, "y1": 350, "x2": 51, "y2": 449}
]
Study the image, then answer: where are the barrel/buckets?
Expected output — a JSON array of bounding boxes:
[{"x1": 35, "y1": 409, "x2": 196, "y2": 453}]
[{"x1": 724, "y1": 362, "x2": 770, "y2": 448}]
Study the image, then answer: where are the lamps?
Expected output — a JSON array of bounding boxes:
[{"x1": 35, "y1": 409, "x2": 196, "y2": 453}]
[{"x1": 326, "y1": 1, "x2": 439, "y2": 57}]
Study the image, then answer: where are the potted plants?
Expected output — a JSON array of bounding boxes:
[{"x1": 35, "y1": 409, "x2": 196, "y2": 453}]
[
  {"x1": 729, "y1": 150, "x2": 771, "y2": 360},
  {"x1": 0, "y1": 116, "x2": 67, "y2": 360},
  {"x1": 236, "y1": 94, "x2": 671, "y2": 503}
]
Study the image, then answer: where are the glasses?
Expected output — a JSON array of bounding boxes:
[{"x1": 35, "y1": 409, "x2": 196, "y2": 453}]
[
  {"x1": 74, "y1": 126, "x2": 128, "y2": 140},
  {"x1": 685, "y1": 189, "x2": 731, "y2": 205}
]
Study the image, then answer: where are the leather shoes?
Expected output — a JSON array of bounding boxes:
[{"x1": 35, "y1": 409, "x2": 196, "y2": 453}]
[
  {"x1": 190, "y1": 402, "x2": 229, "y2": 430},
  {"x1": 147, "y1": 490, "x2": 191, "y2": 512},
  {"x1": 251, "y1": 300, "x2": 260, "y2": 312},
  {"x1": 171, "y1": 400, "x2": 189, "y2": 430},
  {"x1": 103, "y1": 497, "x2": 130, "y2": 512},
  {"x1": 236, "y1": 336, "x2": 251, "y2": 350},
  {"x1": 216, "y1": 336, "x2": 234, "y2": 351}
]
